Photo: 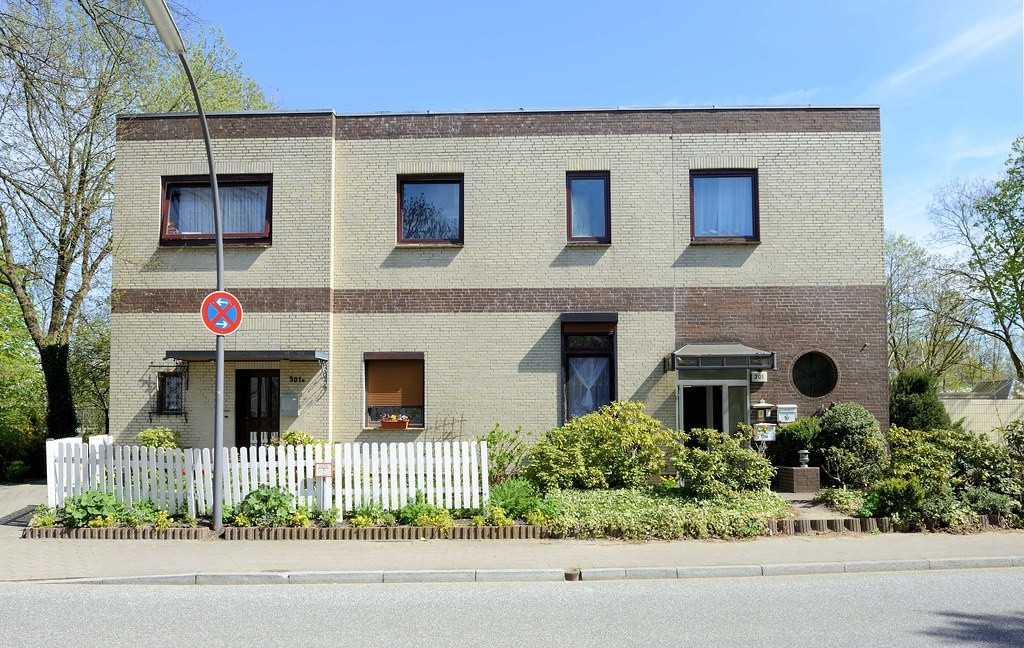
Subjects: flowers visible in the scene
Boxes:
[379,413,409,422]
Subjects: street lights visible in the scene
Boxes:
[142,0,225,534]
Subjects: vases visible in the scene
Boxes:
[381,420,409,430]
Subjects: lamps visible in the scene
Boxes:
[664,357,672,373]
[796,449,811,468]
